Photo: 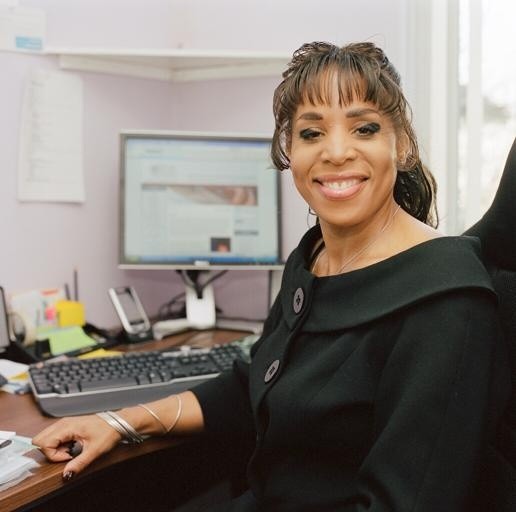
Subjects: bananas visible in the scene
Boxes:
[26,340,250,420]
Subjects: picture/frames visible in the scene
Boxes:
[0,327,252,512]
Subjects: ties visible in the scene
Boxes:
[116,129,287,334]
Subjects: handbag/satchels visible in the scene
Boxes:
[106,411,145,444]
[95,411,142,444]
[137,403,169,435]
[167,394,182,433]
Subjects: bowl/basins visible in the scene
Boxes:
[337,202,403,275]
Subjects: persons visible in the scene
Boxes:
[28,39,516,512]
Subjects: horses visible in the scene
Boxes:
[109,286,151,334]
